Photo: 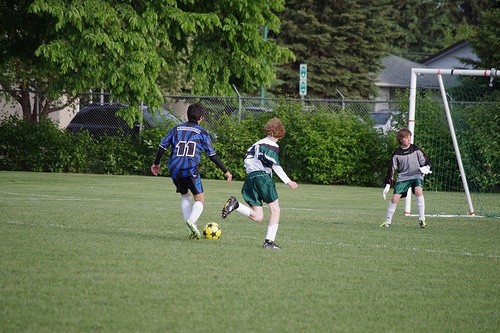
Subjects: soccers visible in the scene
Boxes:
[203,222,221,240]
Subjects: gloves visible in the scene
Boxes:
[418,165,432,175]
[383,184,391,200]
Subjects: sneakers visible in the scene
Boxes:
[221,196,238,220]
[263,239,281,250]
[418,220,426,228]
[379,222,391,227]
[186,219,200,240]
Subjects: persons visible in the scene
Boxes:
[378,128,434,228]
[221,116,298,249]
[150,102,233,240]
